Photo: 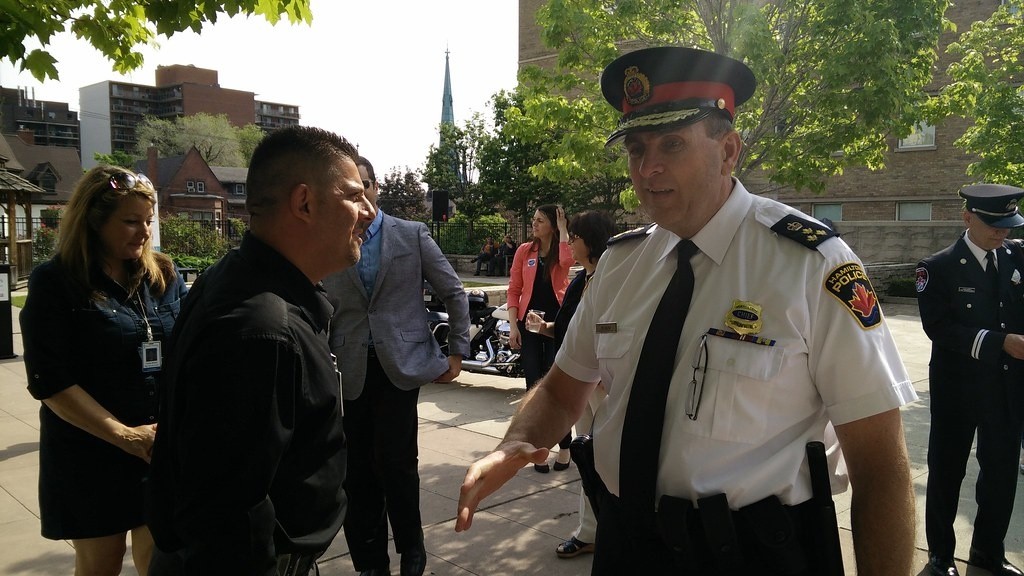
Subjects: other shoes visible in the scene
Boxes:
[473,271,479,275]
[471,258,478,263]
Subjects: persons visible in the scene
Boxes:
[455,46,914,576]
[19,164,190,576]
[322,156,470,576]
[913,184,1024,576]
[554,209,621,559]
[145,128,376,576]
[471,235,516,277]
[506,205,575,473]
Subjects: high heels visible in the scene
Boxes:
[535,462,550,473]
[553,453,571,471]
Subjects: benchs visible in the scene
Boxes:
[477,248,516,276]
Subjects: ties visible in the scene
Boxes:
[985,251,998,289]
[618,240,700,521]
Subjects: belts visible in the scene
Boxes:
[367,345,377,357]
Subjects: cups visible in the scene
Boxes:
[528,310,546,333]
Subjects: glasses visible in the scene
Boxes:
[362,179,374,189]
[685,334,708,420]
[568,233,584,245]
[87,172,154,209]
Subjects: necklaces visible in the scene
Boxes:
[538,249,549,267]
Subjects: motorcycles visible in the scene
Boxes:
[424,287,524,378]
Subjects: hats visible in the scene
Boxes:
[600,47,756,149]
[959,184,1024,228]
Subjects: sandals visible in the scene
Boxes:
[556,536,596,557]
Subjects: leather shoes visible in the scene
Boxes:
[966,556,1024,576]
[358,563,390,576]
[928,546,959,576]
[400,528,426,576]
